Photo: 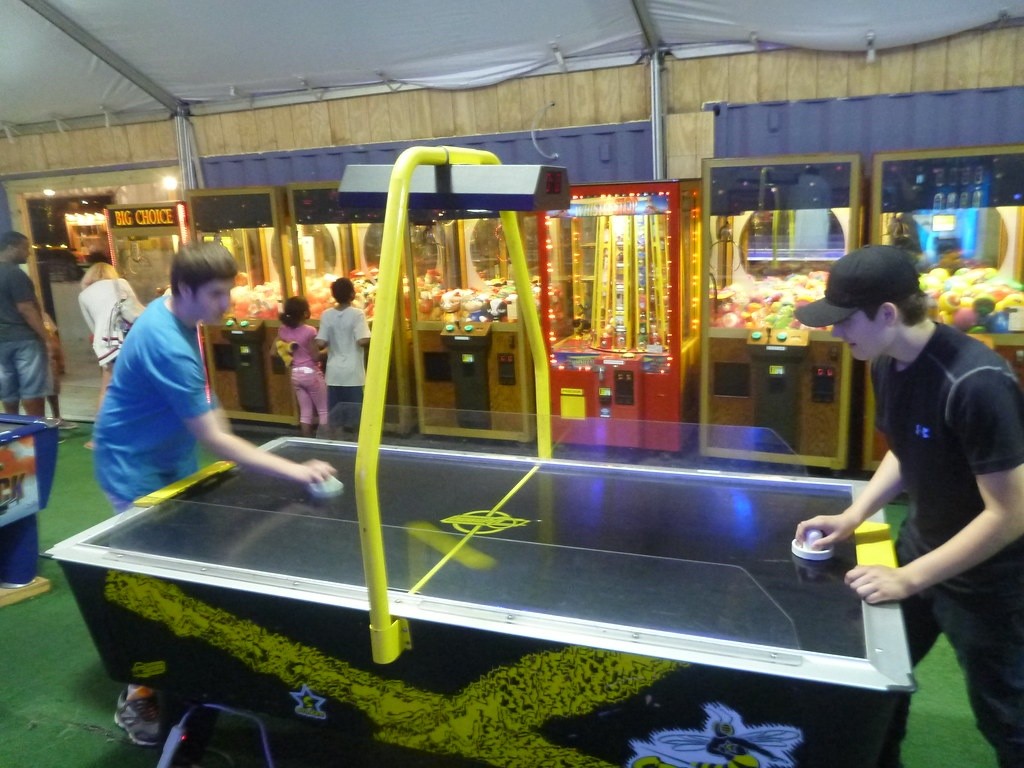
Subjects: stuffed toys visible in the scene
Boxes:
[920,267,1024,332]
[308,267,379,317]
[275,341,299,367]
[715,270,834,332]
[230,281,297,319]
[401,270,541,323]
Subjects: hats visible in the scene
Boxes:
[793,244,921,327]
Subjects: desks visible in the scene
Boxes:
[38,434,918,768]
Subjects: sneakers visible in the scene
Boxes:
[114,688,164,745]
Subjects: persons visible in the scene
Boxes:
[930,237,971,273]
[78,262,136,450]
[93,243,336,746]
[314,277,371,440]
[269,296,329,438]
[794,245,1023,768]
[0,231,61,425]
